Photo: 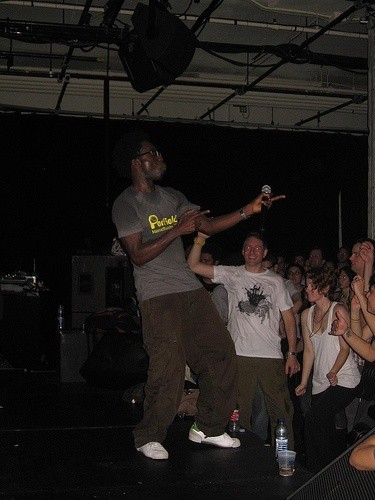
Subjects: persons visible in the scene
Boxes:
[188,232,301,448]
[264,238,375,472]
[96,296,141,334]
[111,138,286,460]
[199,248,245,325]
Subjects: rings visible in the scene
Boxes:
[355,278,357,280]
[269,199,270,201]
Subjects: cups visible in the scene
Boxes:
[277,450,296,477]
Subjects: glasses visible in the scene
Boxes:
[137,148,162,159]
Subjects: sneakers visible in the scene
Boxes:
[137,441,168,460]
[189,421,241,448]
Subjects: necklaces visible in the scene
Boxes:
[313,300,332,324]
[309,300,326,338]
[342,292,347,297]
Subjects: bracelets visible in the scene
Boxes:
[351,315,362,321]
[329,372,336,375]
[240,209,247,219]
[288,352,295,355]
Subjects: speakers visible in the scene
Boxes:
[118,4,198,92]
[70,255,136,332]
[286,426,375,500]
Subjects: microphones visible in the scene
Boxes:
[259,185,271,227]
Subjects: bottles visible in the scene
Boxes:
[275,418,288,462]
[229,406,240,433]
